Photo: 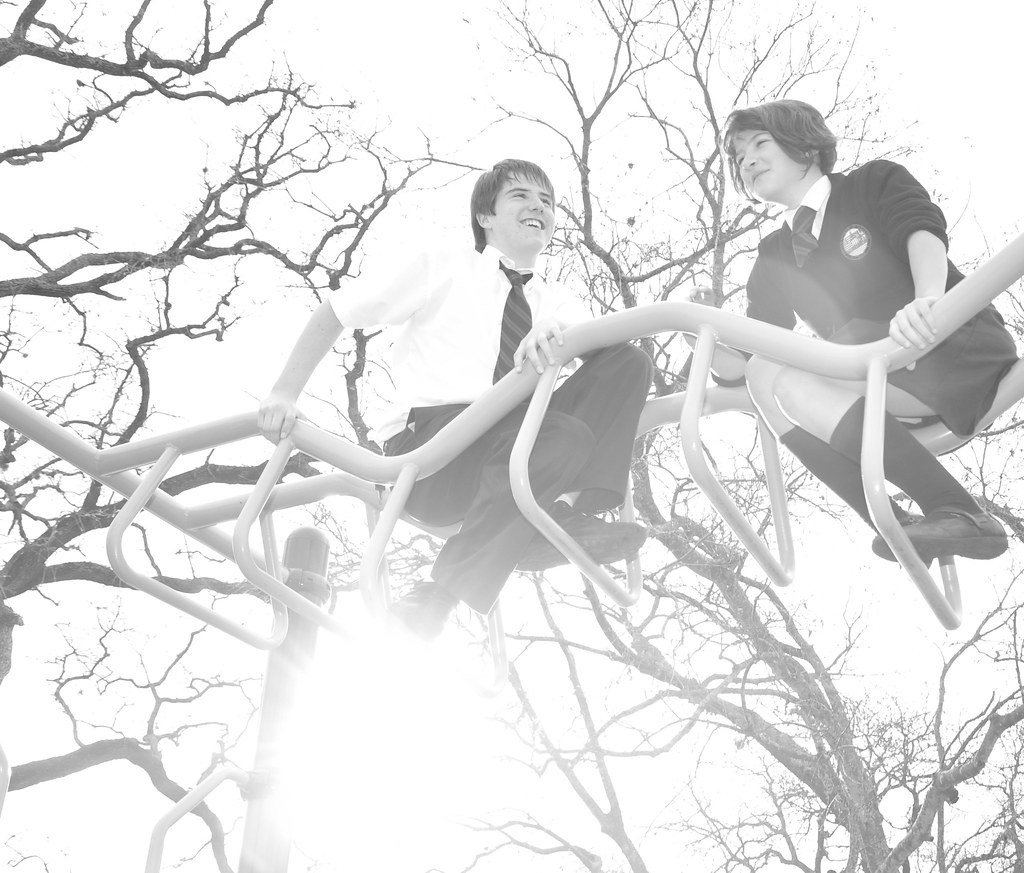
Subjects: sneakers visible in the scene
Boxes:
[516,508,647,571]
[898,511,925,527]
[872,506,1009,564]
[390,581,460,640]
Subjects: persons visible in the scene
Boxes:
[259,159,654,629]
[682,100,1018,566]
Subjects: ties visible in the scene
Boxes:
[790,205,819,268]
[492,260,534,386]
